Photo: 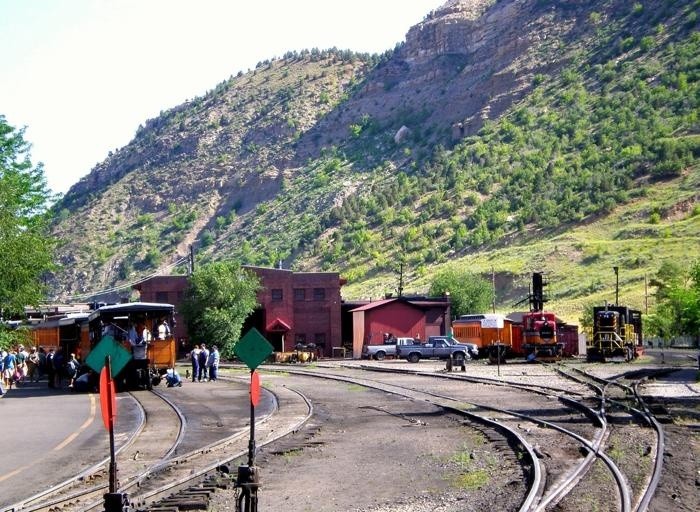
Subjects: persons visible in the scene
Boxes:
[190,345,204,382]
[198,343,209,382]
[208,344,220,382]
[166,368,182,387]
[0,340,94,394]
[100,317,171,347]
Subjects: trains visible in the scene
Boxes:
[451,313,514,356]
[28,299,175,391]
[584,305,643,364]
[521,310,567,363]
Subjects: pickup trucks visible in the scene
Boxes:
[368,334,480,364]
